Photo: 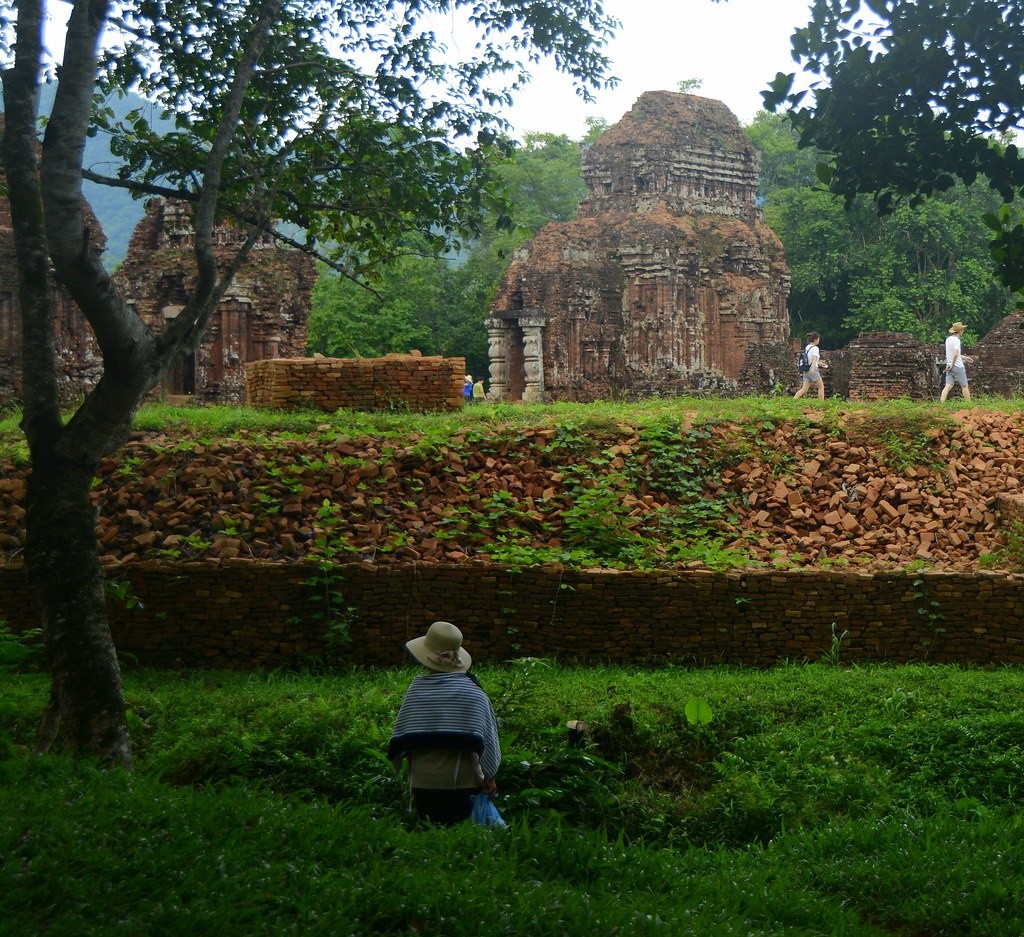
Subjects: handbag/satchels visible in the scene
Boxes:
[472,793,505,829]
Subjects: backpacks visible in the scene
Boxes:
[797,345,814,371]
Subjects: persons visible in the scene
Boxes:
[464,375,474,396]
[472,376,487,400]
[939,322,974,403]
[382,621,502,829]
[793,331,829,400]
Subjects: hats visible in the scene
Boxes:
[464,374,474,385]
[406,621,472,672]
[948,322,969,333]
